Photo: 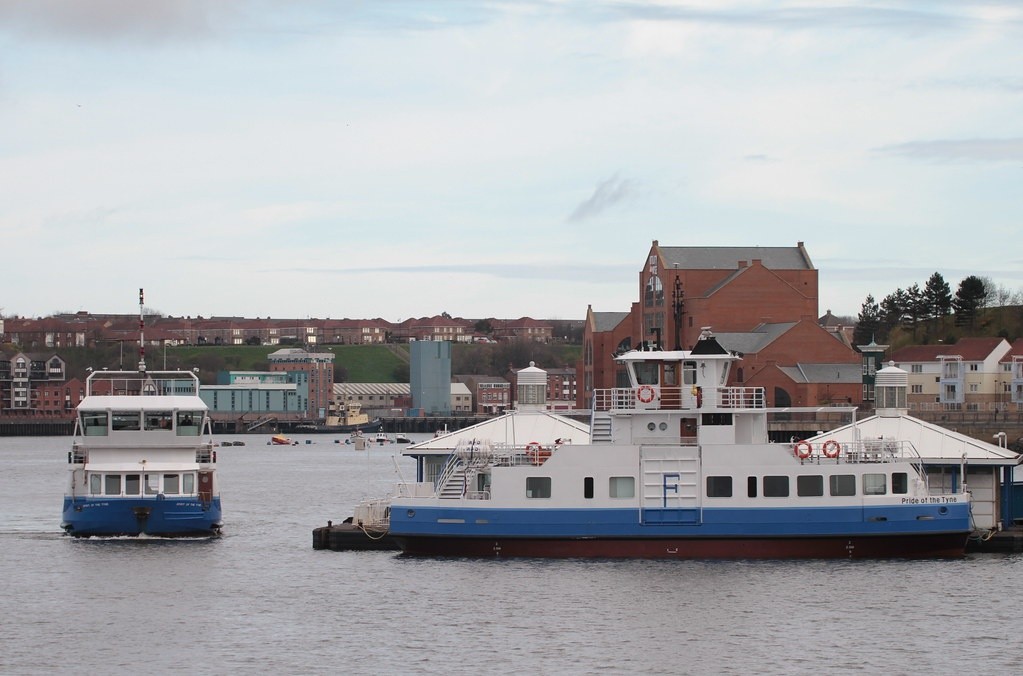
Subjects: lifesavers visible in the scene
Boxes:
[636,385,655,403]
[794,440,813,458]
[822,439,840,457]
[525,441,543,460]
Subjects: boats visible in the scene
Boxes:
[59,288,221,545]
[376,433,387,442]
[313,327,971,560]
[293,401,383,433]
[396,432,411,443]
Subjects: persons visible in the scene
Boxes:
[181,415,192,426]
